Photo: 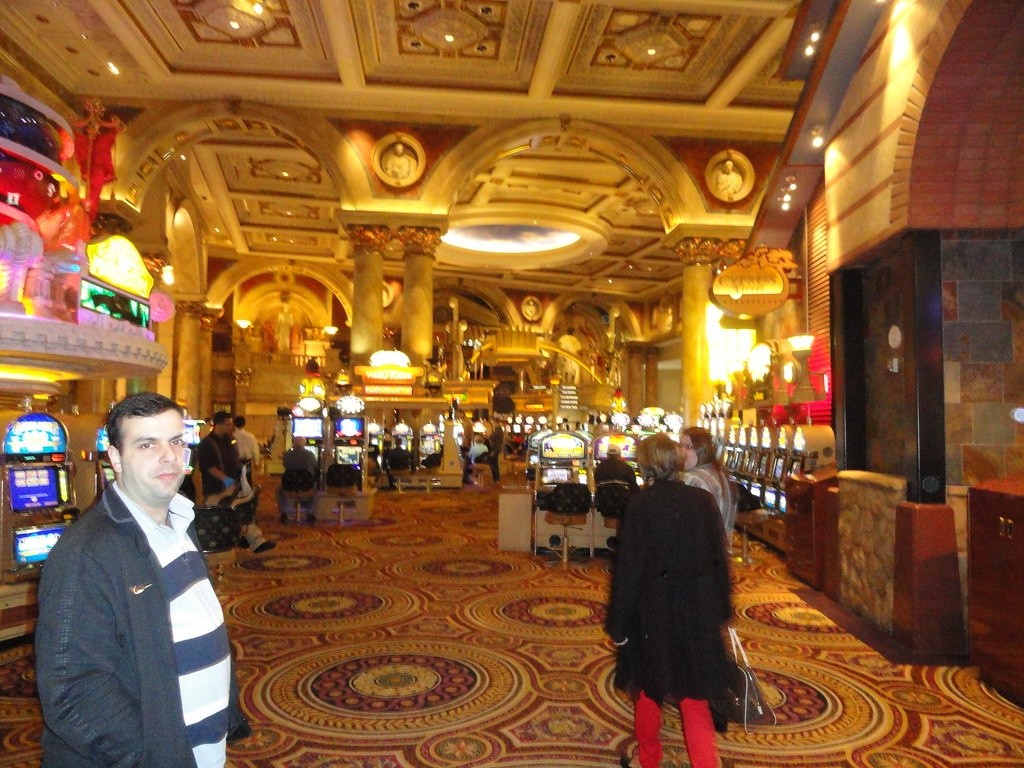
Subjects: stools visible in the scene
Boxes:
[732,482,764,564]
[391,469,407,495]
[470,463,490,488]
[419,453,442,497]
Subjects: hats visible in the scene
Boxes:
[607,445,621,454]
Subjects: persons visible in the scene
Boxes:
[199,411,276,553]
[36,391,252,768]
[606,427,738,768]
[594,445,640,519]
[367,418,523,491]
[280,435,320,523]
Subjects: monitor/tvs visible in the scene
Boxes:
[369,436,442,456]
[8,466,59,511]
[293,417,323,437]
[292,444,320,463]
[540,467,573,484]
[335,418,363,436]
[724,447,802,480]
[335,445,362,464]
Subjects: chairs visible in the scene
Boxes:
[326,464,363,527]
[594,483,630,529]
[536,483,592,571]
[282,467,317,526]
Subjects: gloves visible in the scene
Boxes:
[224,476,237,489]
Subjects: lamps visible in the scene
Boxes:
[142,252,175,285]
[297,375,325,413]
[789,333,815,360]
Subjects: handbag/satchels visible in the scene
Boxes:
[727,627,777,735]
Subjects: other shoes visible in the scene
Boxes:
[281,513,287,522]
[238,536,250,549]
[253,542,276,553]
[620,756,634,768]
[307,514,317,522]
